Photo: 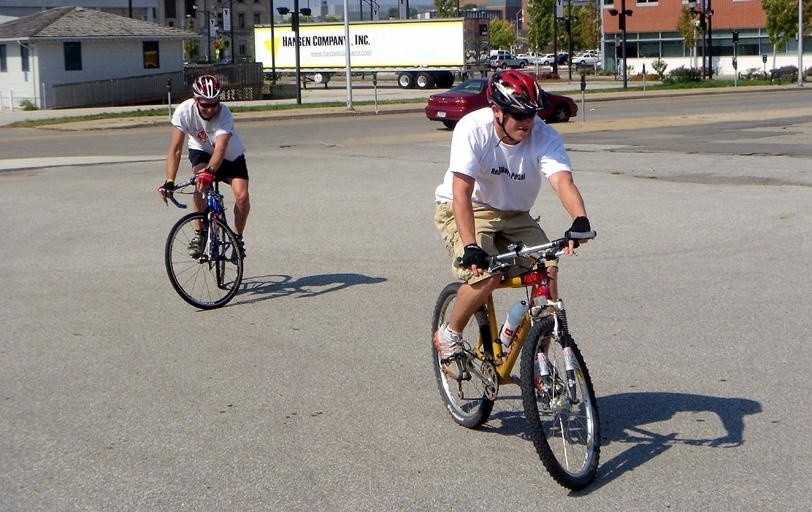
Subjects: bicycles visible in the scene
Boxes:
[158,171,245,309]
[431,232,601,495]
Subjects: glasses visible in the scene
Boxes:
[509,108,540,123]
[198,100,220,109]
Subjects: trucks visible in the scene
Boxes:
[216,17,482,89]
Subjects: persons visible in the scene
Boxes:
[163,74,251,265]
[432,67,592,404]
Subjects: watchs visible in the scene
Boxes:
[206,167,216,173]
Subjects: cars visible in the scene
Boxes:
[489,48,602,75]
[424,76,580,135]
[182,58,212,85]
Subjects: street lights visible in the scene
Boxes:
[688,0,714,82]
[562,0,579,82]
[609,0,634,90]
[277,0,309,104]
[182,1,237,65]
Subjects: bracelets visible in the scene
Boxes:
[167,179,175,184]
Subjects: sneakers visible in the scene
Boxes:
[189,229,209,258]
[433,322,473,381]
[535,355,563,396]
[230,234,246,263]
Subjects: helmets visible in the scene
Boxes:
[486,70,544,114]
[192,74,223,102]
